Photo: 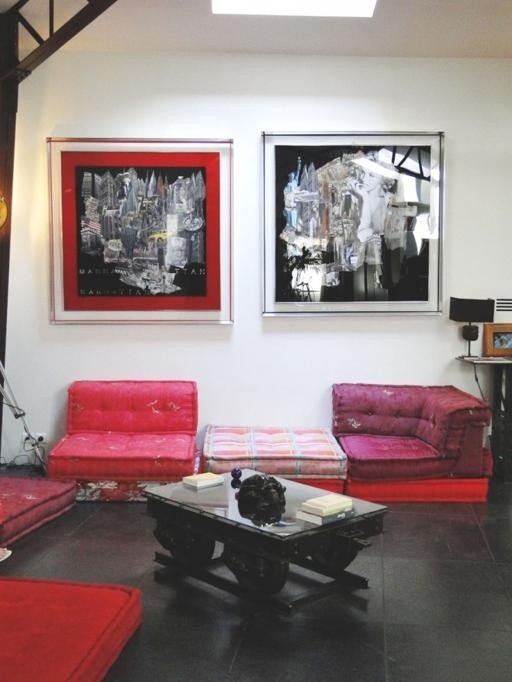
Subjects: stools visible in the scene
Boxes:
[202,427,348,495]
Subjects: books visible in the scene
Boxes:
[463,356,512,364]
[182,472,224,488]
[292,494,355,524]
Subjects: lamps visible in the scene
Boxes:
[449,296,494,358]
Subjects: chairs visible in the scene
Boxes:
[334,382,492,503]
[46,380,199,503]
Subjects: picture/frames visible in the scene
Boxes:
[48,138,235,324]
[482,323,511,358]
[262,132,444,314]
